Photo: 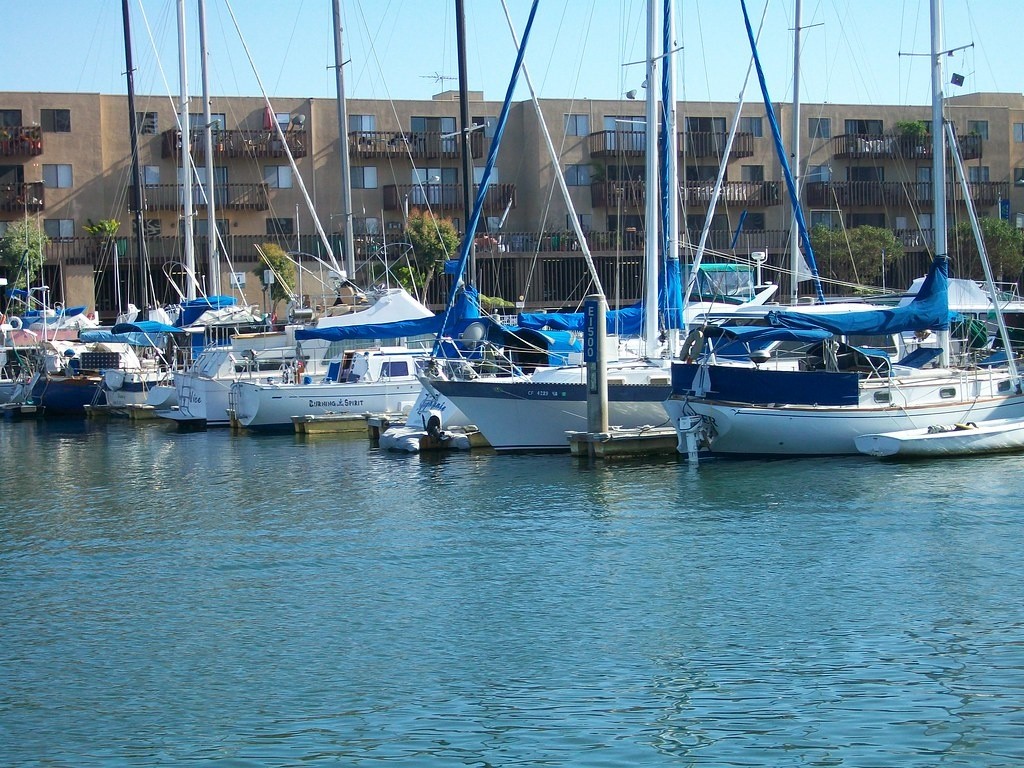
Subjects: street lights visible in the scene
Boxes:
[402,175,438,252]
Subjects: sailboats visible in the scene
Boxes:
[0,0,1024,459]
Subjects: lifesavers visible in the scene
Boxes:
[5,315,23,331]
[678,330,704,363]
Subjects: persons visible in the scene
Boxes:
[421,414,452,442]
